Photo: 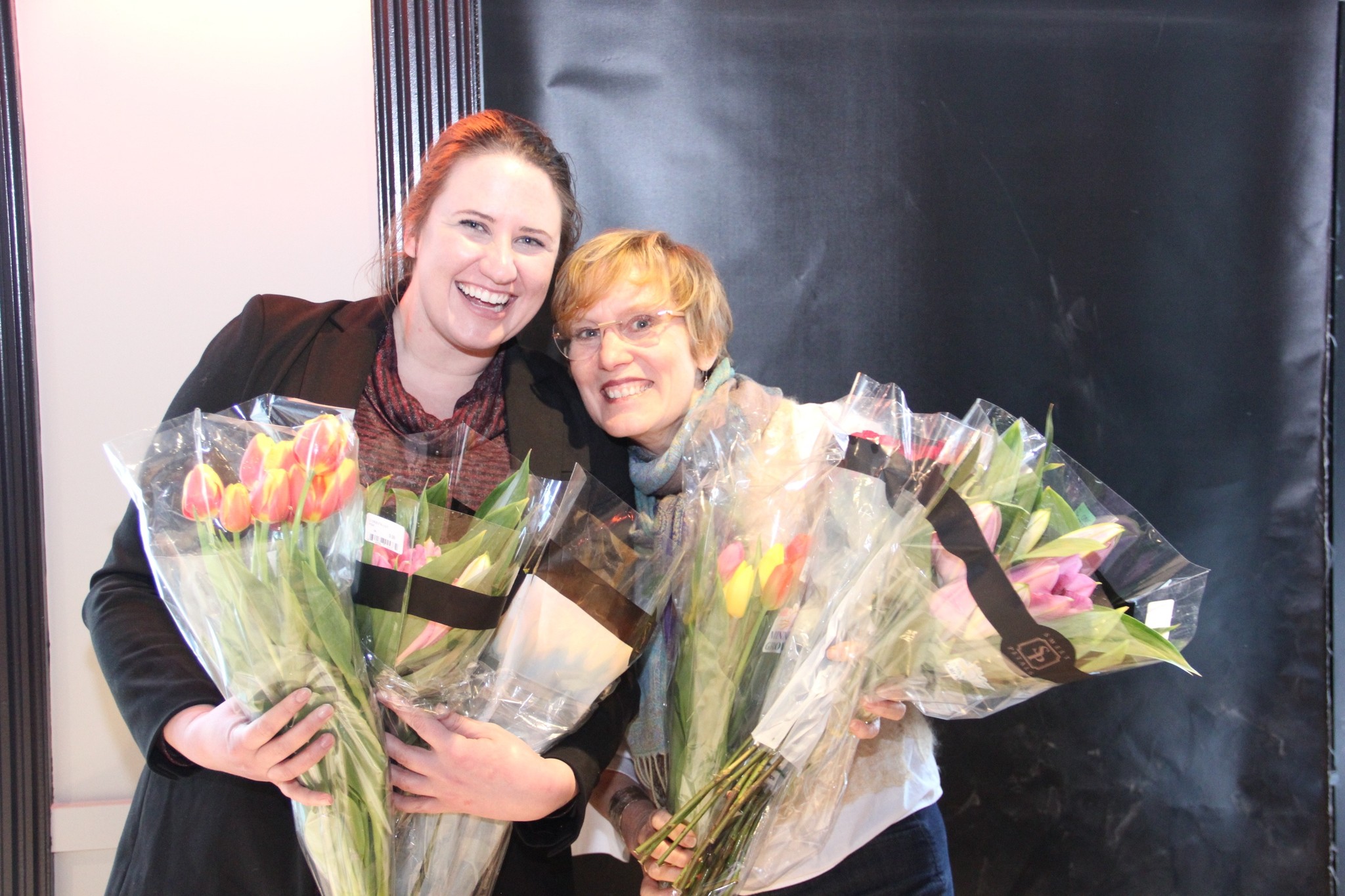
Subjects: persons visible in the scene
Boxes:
[81,111,643,896]
[551,230,955,896]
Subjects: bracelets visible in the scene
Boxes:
[606,785,651,840]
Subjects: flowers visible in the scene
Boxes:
[99,368,1210,896]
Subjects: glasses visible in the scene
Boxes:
[552,305,688,362]
